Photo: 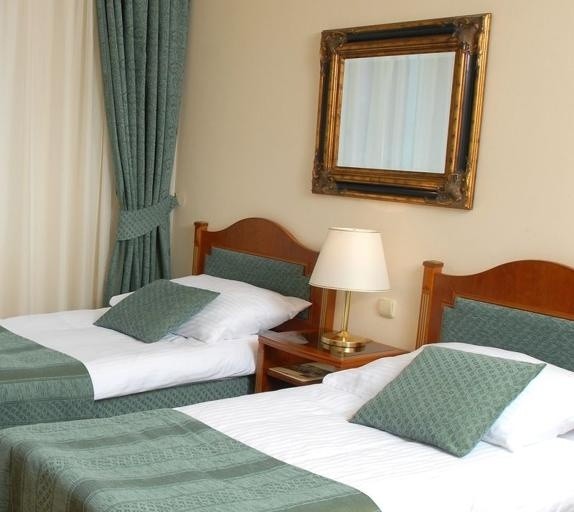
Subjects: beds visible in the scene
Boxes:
[0,215,337,431]
[0,254,574,512]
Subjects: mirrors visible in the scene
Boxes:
[310,11,494,212]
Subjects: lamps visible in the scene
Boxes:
[307,225,393,350]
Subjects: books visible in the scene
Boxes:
[268,362,336,381]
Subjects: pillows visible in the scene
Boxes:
[346,346,549,460]
[91,277,222,345]
[108,272,314,345]
[320,339,574,455]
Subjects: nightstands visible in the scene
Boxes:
[252,328,412,395]
